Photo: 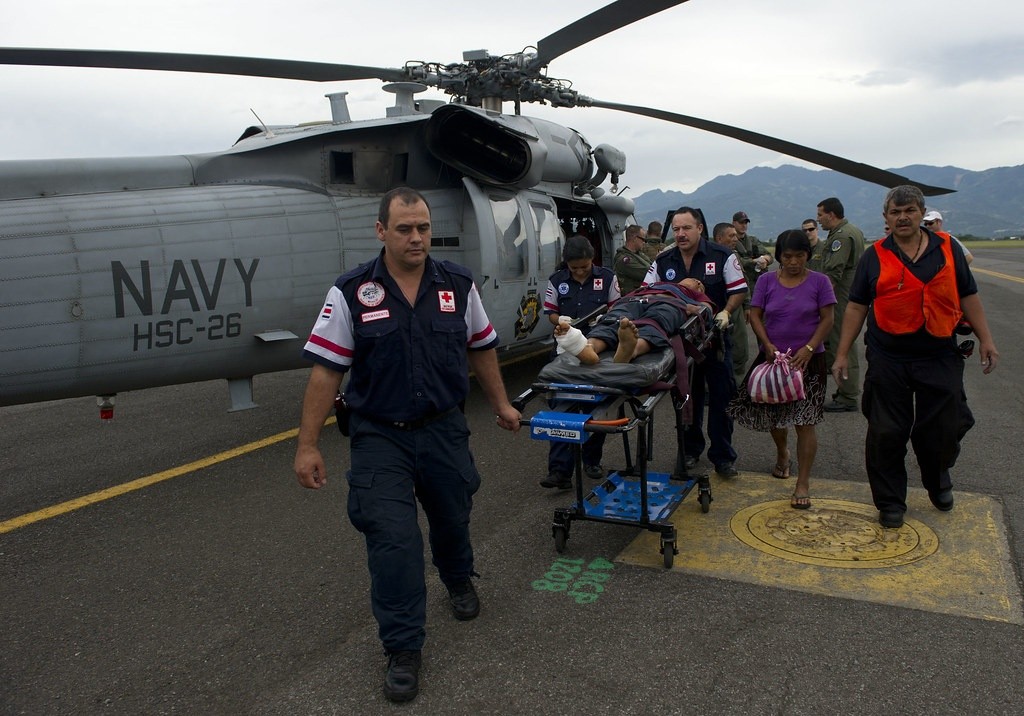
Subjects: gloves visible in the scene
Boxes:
[558,315,573,325]
[715,310,730,330]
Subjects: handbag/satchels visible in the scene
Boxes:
[748,347,805,403]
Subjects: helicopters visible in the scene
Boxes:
[0,0,961,422]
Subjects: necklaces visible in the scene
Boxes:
[778,269,806,286]
[893,230,923,289]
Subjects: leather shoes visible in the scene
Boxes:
[879,510,904,528]
[540,473,572,490]
[714,460,738,476]
[449,575,480,621]
[583,463,602,479]
[383,648,421,703]
[928,485,953,511]
[684,454,699,468]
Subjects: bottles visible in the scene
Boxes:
[755,256,765,272]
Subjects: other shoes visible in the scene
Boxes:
[822,400,858,412]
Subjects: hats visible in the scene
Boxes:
[922,210,943,221]
[733,211,750,223]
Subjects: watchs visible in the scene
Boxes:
[807,345,815,354]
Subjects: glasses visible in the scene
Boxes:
[635,235,645,242]
[885,227,890,230]
[924,220,938,226]
[802,226,816,233]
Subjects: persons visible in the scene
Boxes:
[725,230,837,509]
[802,219,825,272]
[293,187,523,701]
[613,222,667,300]
[553,277,717,366]
[538,236,621,491]
[883,210,973,267]
[713,222,751,385]
[817,198,865,414]
[731,211,775,351]
[830,184,998,528]
[640,205,749,478]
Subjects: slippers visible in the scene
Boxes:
[791,494,811,509]
[772,458,792,478]
